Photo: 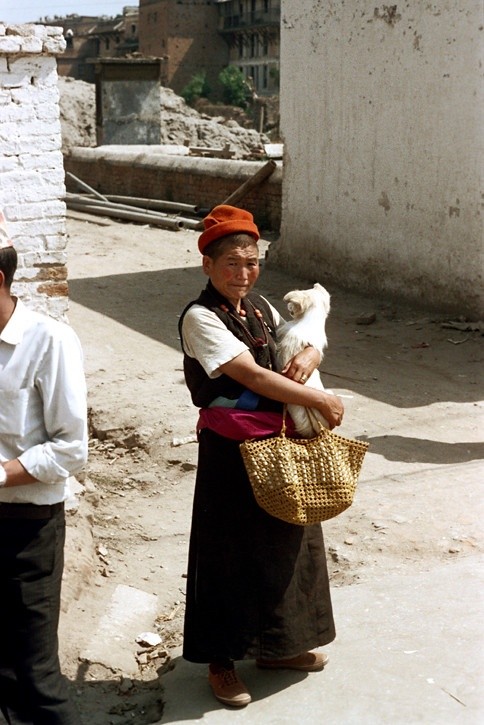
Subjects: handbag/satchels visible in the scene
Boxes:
[239,397,369,526]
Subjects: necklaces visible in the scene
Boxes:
[220,297,268,348]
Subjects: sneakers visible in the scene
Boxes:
[207,666,250,707]
[255,651,328,672]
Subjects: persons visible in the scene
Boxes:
[177,205,344,706]
[0,212,89,725]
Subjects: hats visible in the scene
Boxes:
[197,203,261,254]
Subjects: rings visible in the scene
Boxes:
[301,374,307,381]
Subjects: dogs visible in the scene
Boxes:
[274,283,330,438]
